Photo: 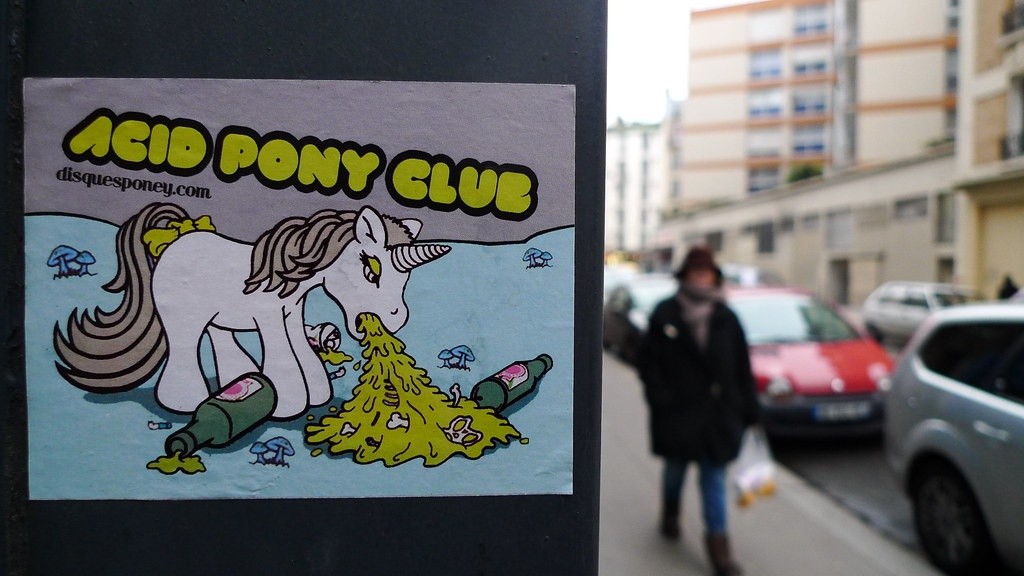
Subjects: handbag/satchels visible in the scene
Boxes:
[732,427,782,510]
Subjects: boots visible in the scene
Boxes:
[704,533,744,576]
[657,499,682,542]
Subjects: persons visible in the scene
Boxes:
[638,247,766,576]
[998,276,1018,299]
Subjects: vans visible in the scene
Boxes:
[719,285,896,461]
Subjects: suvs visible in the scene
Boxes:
[863,283,971,348]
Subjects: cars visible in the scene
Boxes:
[886,303,1023,576]
[604,271,679,366]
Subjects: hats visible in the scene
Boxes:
[673,246,725,290]
